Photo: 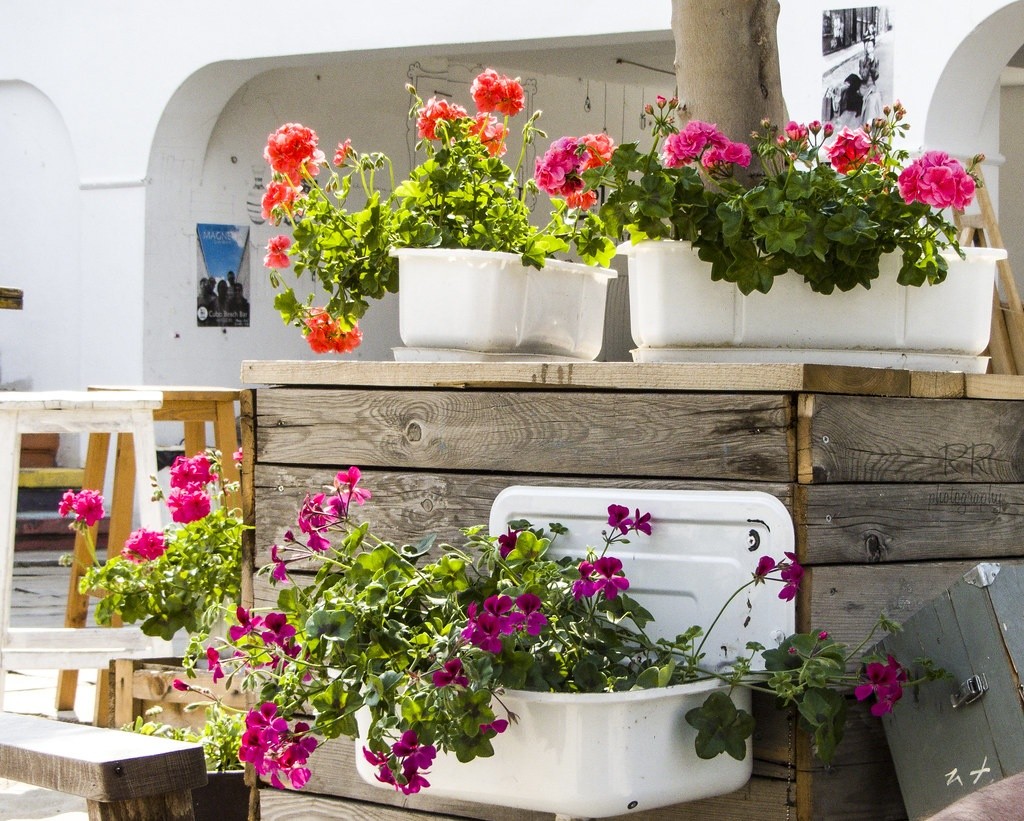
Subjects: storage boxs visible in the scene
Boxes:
[239,355,1024,820]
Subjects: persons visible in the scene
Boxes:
[829,12,888,128]
[198,270,250,324]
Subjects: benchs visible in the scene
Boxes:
[0,717,208,821]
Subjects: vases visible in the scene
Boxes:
[354,483,796,819]
[387,248,620,364]
[613,238,1007,373]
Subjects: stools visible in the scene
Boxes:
[0,385,242,732]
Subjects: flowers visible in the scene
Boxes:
[170,461,957,798]
[58,445,243,639]
[260,66,619,353]
[535,94,986,295]
